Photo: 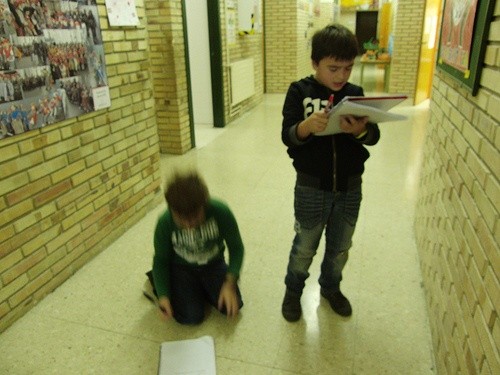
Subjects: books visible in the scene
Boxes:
[157,334,217,375]
[315,94,408,136]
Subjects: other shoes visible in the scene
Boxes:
[281,288,303,322]
[145,269,162,296]
[320,285,352,317]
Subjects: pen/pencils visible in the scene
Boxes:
[325,94,334,113]
[142,292,167,316]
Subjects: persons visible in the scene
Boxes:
[141,167,245,326]
[0,1,106,137]
[281,23,380,323]
